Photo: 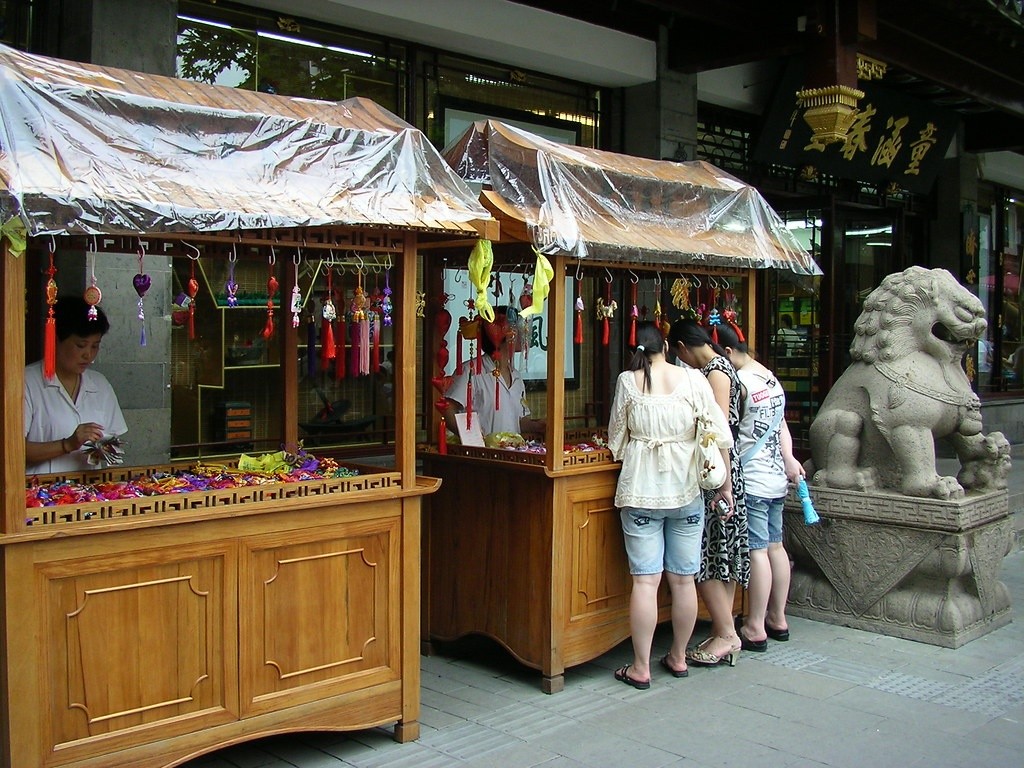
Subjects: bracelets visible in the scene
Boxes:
[62,438,70,454]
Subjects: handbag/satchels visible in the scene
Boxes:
[686,368,727,490]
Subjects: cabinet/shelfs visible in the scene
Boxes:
[417,442,750,694]
[0,473,441,768]
[775,356,820,425]
[172,17,394,461]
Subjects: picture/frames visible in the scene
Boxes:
[434,93,583,393]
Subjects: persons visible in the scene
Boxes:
[609,321,733,690]
[445,306,547,438]
[978,341,1017,382]
[380,351,394,375]
[667,320,750,667]
[771,315,802,355]
[710,324,805,651]
[25,295,128,473]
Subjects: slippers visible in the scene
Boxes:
[736,626,767,652]
[660,651,688,678]
[614,665,650,689]
[764,618,790,641]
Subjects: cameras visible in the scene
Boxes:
[715,500,729,516]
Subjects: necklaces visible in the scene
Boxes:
[56,373,78,399]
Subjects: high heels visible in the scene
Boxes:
[685,631,741,667]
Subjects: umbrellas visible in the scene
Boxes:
[796,475,819,525]
